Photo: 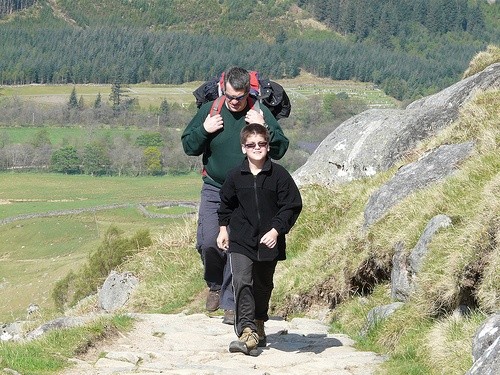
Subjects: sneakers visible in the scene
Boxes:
[223,309,236,324]
[228,318,268,356]
[206,289,222,311]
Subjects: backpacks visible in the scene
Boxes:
[206,67,262,134]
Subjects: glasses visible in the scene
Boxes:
[224,92,248,100]
[244,141,268,148]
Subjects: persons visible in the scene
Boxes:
[216,123,304,356]
[180,66,290,325]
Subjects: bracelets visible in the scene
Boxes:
[262,122,269,129]
